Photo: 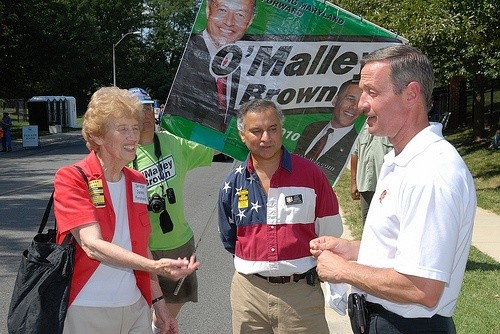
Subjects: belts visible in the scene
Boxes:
[253,266,317,283]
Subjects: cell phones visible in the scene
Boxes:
[353,295,365,333]
[166,187,176,204]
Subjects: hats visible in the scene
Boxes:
[127,88,154,104]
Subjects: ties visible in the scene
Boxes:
[305,128,335,161]
[216,58,227,115]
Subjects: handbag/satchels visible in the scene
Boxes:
[7,165,88,334]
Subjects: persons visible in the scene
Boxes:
[217,99,344,334]
[0,111,12,152]
[164,1,256,132]
[54,86,201,334]
[309,46,477,334]
[469,92,500,155]
[123,88,223,334]
[350,113,395,231]
[293,79,367,181]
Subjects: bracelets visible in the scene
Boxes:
[152,295,164,304]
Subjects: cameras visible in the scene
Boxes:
[147,193,166,213]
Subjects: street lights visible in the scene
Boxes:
[113,32,141,87]
